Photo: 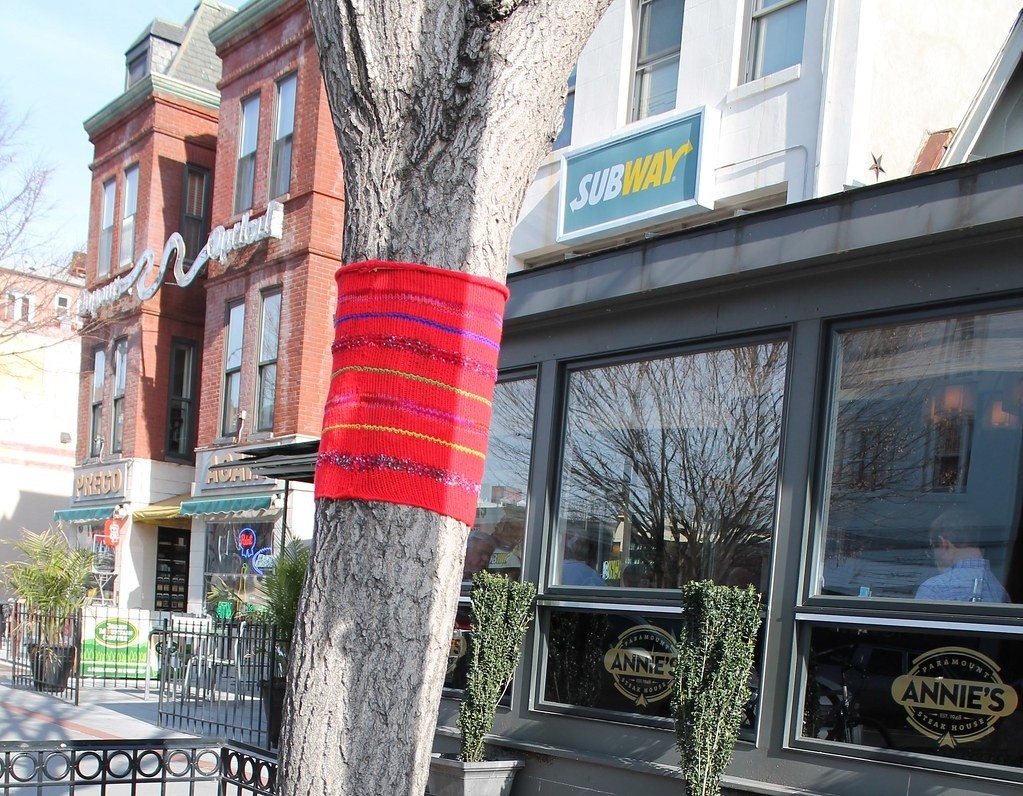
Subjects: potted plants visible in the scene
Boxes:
[1,515,114,693]
[206,518,314,749]
[425,569,539,796]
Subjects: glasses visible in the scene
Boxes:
[924,541,935,559]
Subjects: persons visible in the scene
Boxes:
[463,531,498,575]
[559,525,607,586]
[914,508,1012,604]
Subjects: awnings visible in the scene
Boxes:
[178,494,281,514]
[53,506,119,523]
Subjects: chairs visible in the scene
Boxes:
[183,620,247,708]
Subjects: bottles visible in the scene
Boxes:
[969,577,986,603]
